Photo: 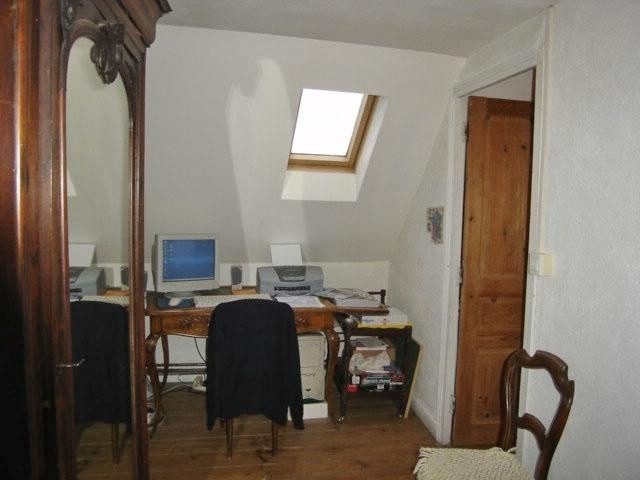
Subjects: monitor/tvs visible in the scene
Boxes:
[151,233,221,299]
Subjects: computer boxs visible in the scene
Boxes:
[297,333,325,402]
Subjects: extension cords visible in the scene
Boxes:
[192,382,206,392]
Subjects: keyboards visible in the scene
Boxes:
[193,294,273,309]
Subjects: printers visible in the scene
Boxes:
[69,266,106,299]
[257,265,324,299]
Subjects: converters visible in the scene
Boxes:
[192,377,202,386]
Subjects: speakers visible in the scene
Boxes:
[231,265,243,290]
[120,264,130,291]
[144,271,148,298]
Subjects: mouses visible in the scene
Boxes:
[168,298,183,307]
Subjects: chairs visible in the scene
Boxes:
[412,348,574,480]
[209,297,291,459]
[71,300,129,467]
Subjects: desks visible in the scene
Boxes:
[68,288,130,308]
[144,291,344,437]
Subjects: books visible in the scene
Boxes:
[354,335,388,352]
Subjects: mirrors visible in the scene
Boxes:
[58,19,136,480]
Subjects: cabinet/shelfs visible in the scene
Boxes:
[335,288,412,420]
